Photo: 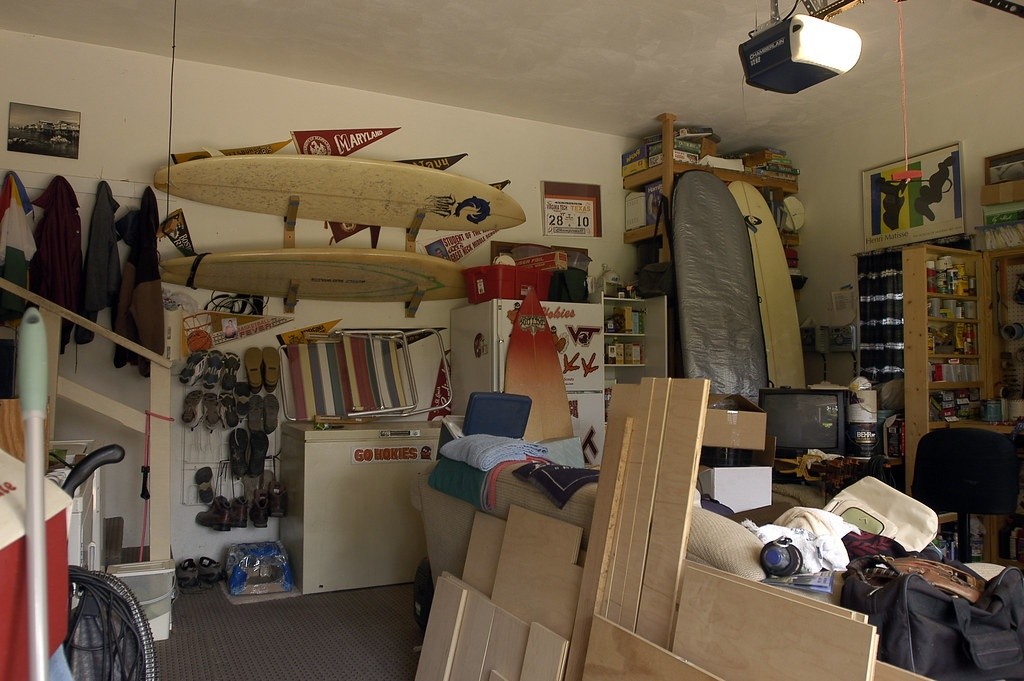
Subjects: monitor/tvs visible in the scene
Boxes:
[758,387,848,457]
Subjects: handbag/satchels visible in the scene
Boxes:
[638,261,675,298]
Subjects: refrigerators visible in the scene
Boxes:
[279,421,443,595]
[450,299,605,469]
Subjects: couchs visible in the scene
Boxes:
[409,463,844,668]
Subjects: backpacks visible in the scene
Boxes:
[842,552,1023,681]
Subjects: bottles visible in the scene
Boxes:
[930,518,1024,564]
[612,336,618,346]
[761,536,833,578]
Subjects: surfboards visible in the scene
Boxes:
[158,247,468,303]
[504,286,574,443]
[154,154,527,232]
[670,170,769,406]
[727,180,806,389]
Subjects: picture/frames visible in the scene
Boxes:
[985,148,1024,185]
[8,102,81,159]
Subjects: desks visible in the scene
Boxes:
[775,458,902,480]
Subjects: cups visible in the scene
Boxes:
[943,300,957,318]
[929,297,940,317]
[928,362,979,381]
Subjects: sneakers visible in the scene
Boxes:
[176,556,224,586]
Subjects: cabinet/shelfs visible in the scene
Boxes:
[591,290,668,426]
[902,243,1024,571]
[623,112,801,301]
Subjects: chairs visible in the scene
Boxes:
[278,328,452,422]
[911,428,1020,563]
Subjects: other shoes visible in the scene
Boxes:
[178,347,287,531]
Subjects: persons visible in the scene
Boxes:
[226,320,235,337]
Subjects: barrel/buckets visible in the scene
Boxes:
[846,422,881,457]
[877,410,904,459]
[847,390,877,422]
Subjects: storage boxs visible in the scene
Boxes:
[0,448,74,681]
[105,559,175,642]
[622,127,799,178]
[975,220,1024,250]
[702,393,776,513]
[462,244,593,306]
[626,181,664,231]
[536,437,585,468]
[980,179,1024,206]
[437,392,532,462]
